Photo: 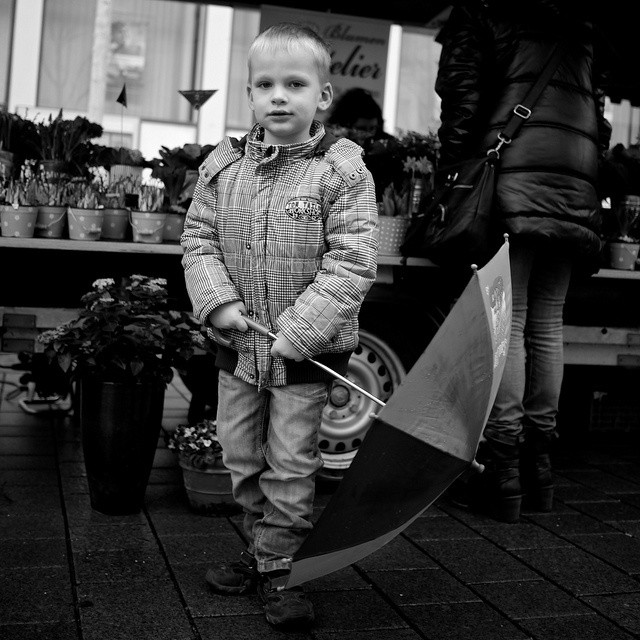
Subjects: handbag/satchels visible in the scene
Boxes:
[427,148,496,267]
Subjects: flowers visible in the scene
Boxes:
[128,176,166,211]
[168,418,224,469]
[401,156,434,179]
[34,273,209,380]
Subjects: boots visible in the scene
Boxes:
[465,447,522,524]
[524,452,555,513]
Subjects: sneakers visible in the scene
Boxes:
[205,551,257,593]
[258,570,314,629]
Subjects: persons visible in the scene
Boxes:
[428,0,607,461]
[178,18,380,631]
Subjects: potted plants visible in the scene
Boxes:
[0,177,38,238]
[606,195,640,270]
[104,145,147,182]
[36,170,66,238]
[27,106,102,172]
[151,144,196,242]
[101,172,132,240]
[0,108,25,177]
[376,181,413,256]
[66,177,106,241]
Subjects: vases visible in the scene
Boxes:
[32,380,207,515]
[408,178,428,213]
[179,461,241,506]
[132,211,167,244]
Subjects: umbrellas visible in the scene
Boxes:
[195,231,516,593]
